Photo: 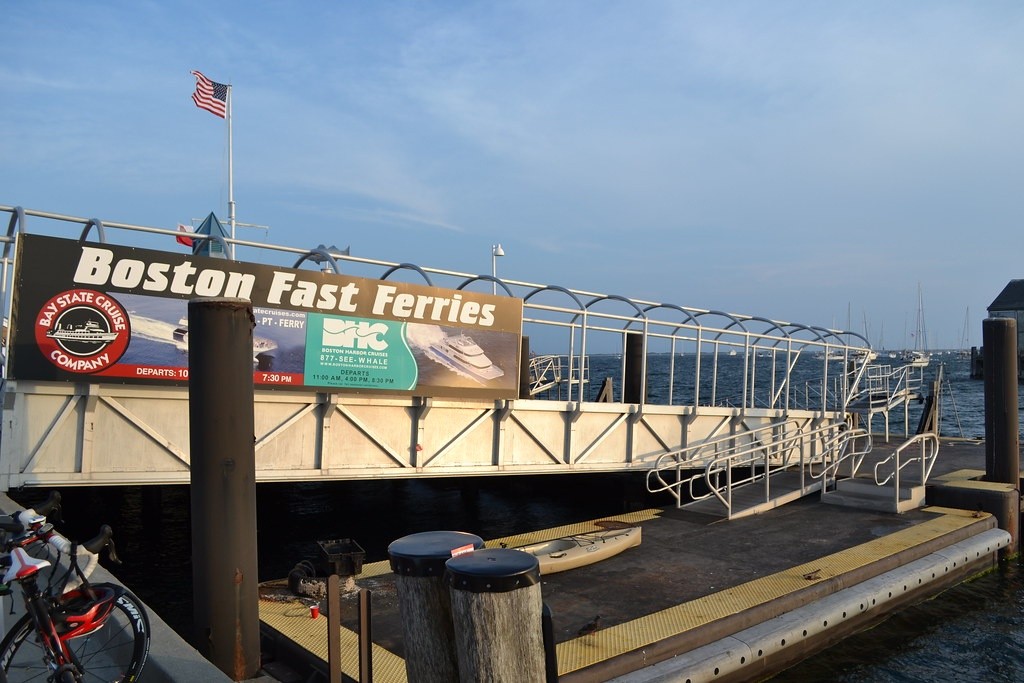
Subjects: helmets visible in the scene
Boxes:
[35,582,127,644]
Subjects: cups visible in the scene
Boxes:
[310,605,319,618]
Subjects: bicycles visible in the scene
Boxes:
[1,491,151,683]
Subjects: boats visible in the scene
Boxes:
[508,526,641,574]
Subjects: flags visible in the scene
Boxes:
[190,70,230,120]
